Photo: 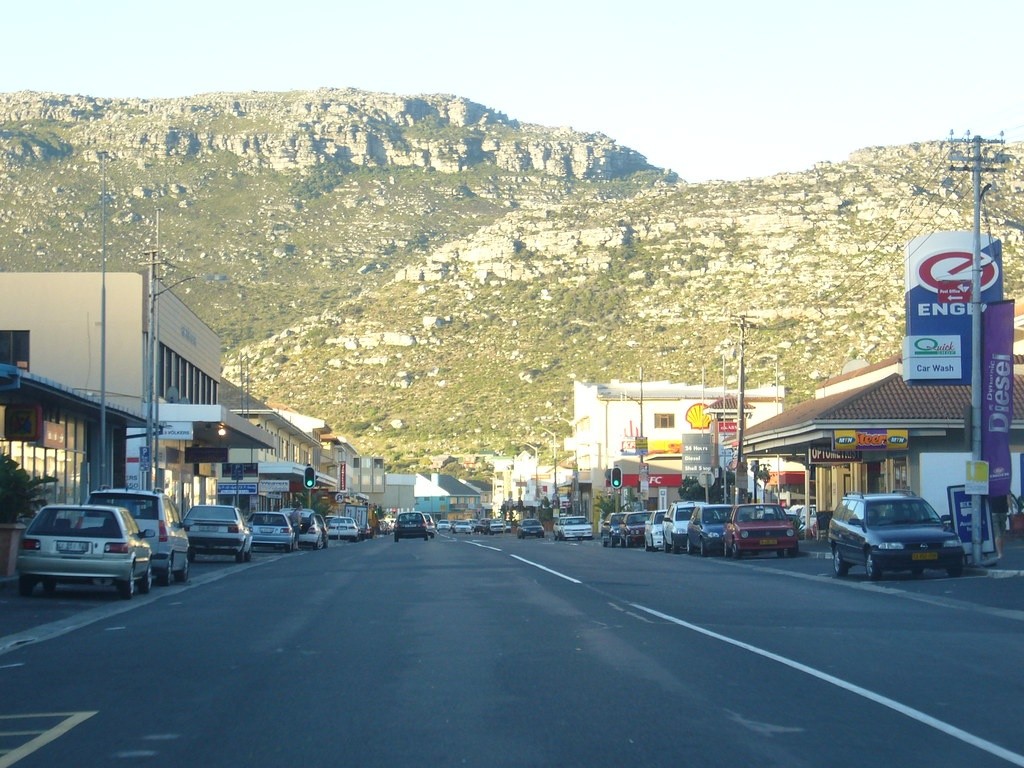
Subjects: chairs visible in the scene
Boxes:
[102,517,119,535]
[54,518,72,533]
[763,513,775,519]
[401,516,406,521]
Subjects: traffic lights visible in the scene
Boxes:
[303,466,315,490]
[610,467,622,489]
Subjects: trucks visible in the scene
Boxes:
[345,504,368,541]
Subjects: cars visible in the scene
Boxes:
[316,513,329,549]
[437,499,800,560]
[827,488,966,581]
[85,484,192,586]
[16,503,153,600]
[393,511,429,542]
[327,516,359,543]
[184,505,253,564]
[246,511,295,553]
[278,507,325,550]
[422,513,435,538]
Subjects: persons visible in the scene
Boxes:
[292,504,303,551]
[989,494,1011,559]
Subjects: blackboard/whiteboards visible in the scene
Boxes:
[816,511,833,531]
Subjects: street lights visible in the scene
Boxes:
[144,274,228,489]
[511,440,539,496]
[528,424,558,493]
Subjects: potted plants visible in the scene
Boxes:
[0,454,60,577]
